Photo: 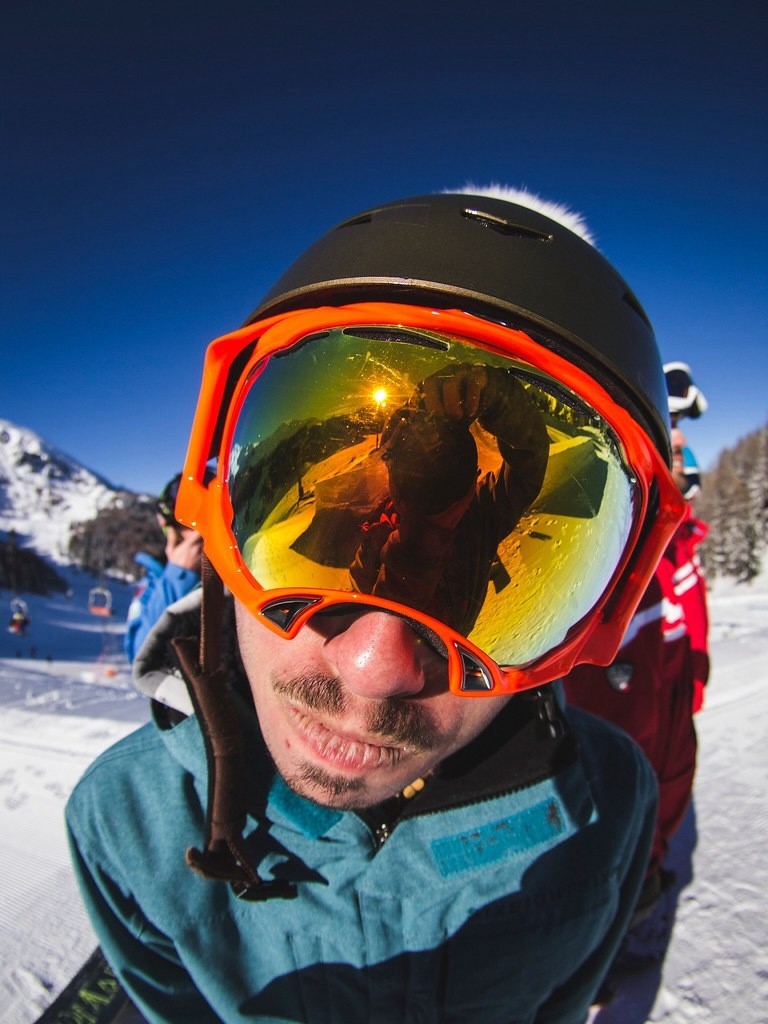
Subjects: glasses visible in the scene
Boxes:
[662,362,708,420]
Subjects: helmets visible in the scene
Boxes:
[156,467,218,527]
[169,191,692,698]
[379,400,482,517]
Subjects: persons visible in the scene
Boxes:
[63,189,696,1024]
[121,361,711,976]
